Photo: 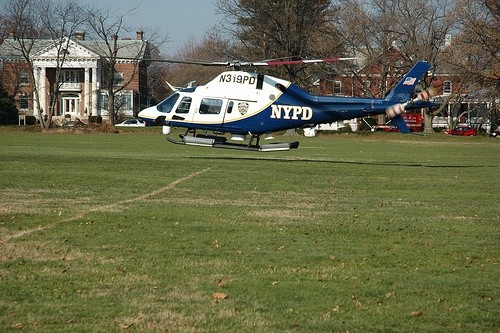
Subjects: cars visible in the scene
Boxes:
[444,126,477,138]
[113,118,147,128]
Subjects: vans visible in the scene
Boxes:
[374,113,427,133]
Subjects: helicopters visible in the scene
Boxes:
[95,51,442,152]
